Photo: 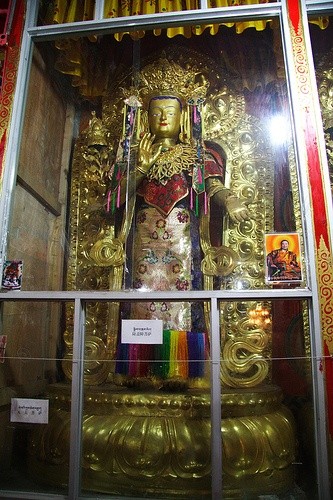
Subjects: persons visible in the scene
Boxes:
[94,58,252,392]
